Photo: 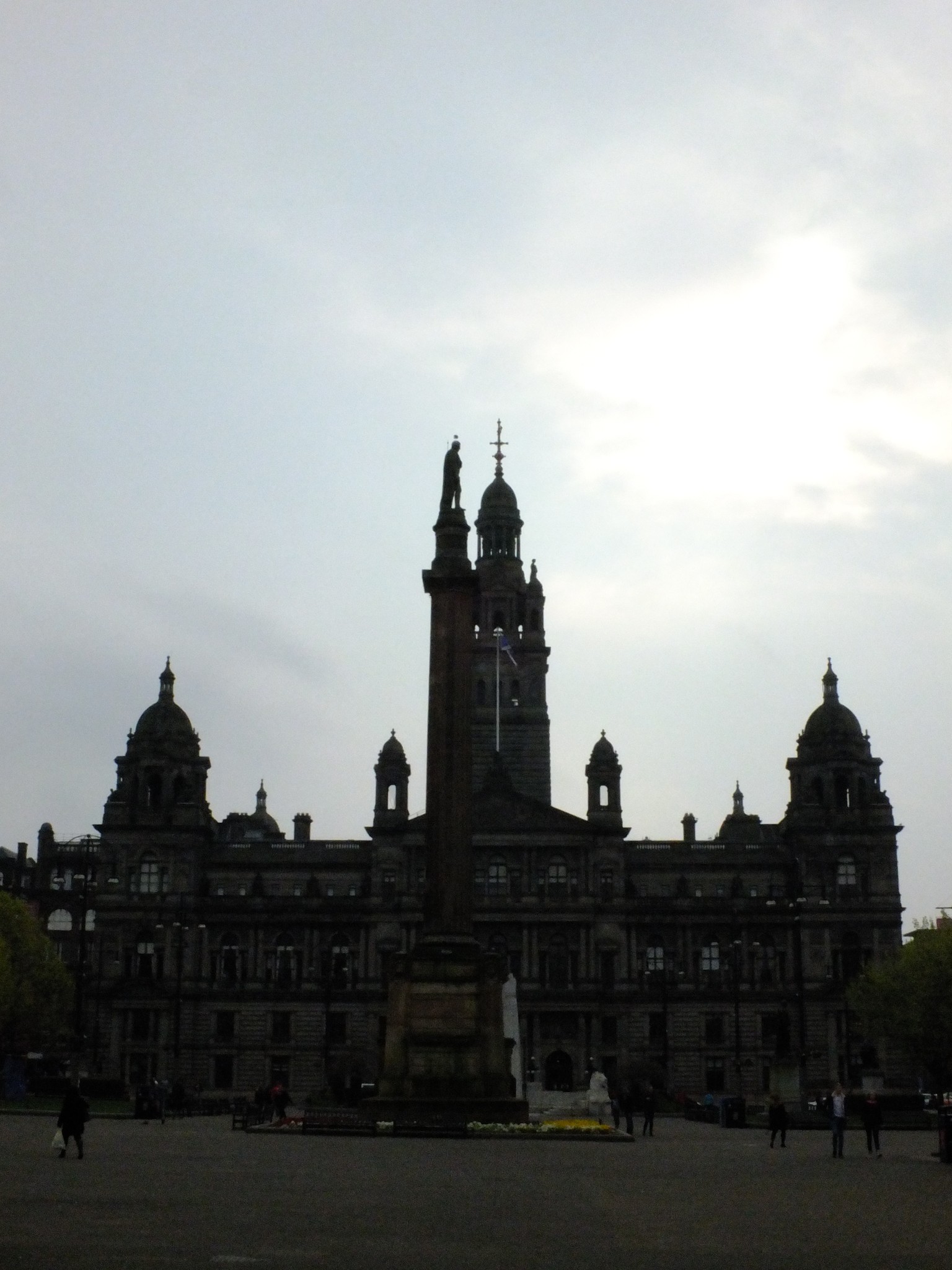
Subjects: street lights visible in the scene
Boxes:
[646,930,668,1104]
[137,924,156,1085]
[72,902,96,1088]
[701,935,721,1099]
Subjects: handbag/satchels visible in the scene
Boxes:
[51,1128,66,1150]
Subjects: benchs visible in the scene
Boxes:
[179,1097,251,1117]
[231,1105,273,1131]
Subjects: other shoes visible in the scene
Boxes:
[781,1144,787,1148]
[932,1149,940,1156]
[875,1149,881,1155]
[144,1120,149,1124]
[643,1130,646,1135]
[649,1131,653,1137]
[78,1152,84,1159]
[58,1151,66,1158]
[162,1120,167,1126]
[770,1142,773,1148]
[939,1157,952,1163]
[867,1146,873,1154]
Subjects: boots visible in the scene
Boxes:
[832,1150,843,1158]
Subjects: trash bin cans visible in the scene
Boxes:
[936,1106,952,1164]
[134,1087,161,1118]
[719,1097,746,1128]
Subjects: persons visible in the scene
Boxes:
[825,1082,853,1160]
[255,1082,286,1123]
[610,1079,635,1136]
[641,1085,656,1137]
[57,1087,89,1160]
[767,1092,789,1149]
[861,1088,883,1157]
